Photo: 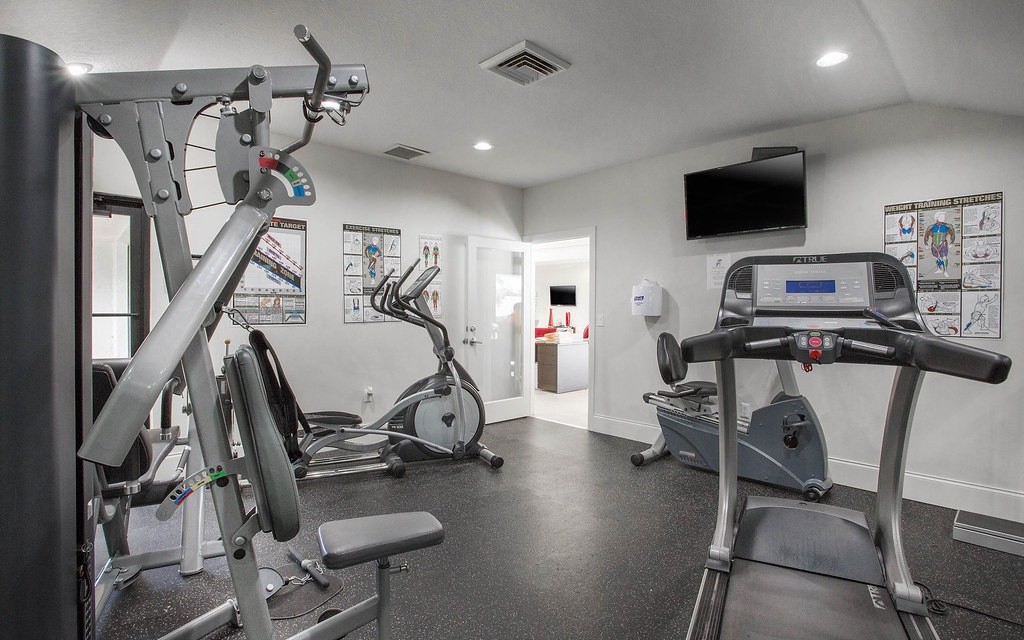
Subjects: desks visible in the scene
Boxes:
[536,342,589,394]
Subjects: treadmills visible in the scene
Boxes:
[679,249,1016,639]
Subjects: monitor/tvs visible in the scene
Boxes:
[550,286,576,306]
[684,149,807,240]
